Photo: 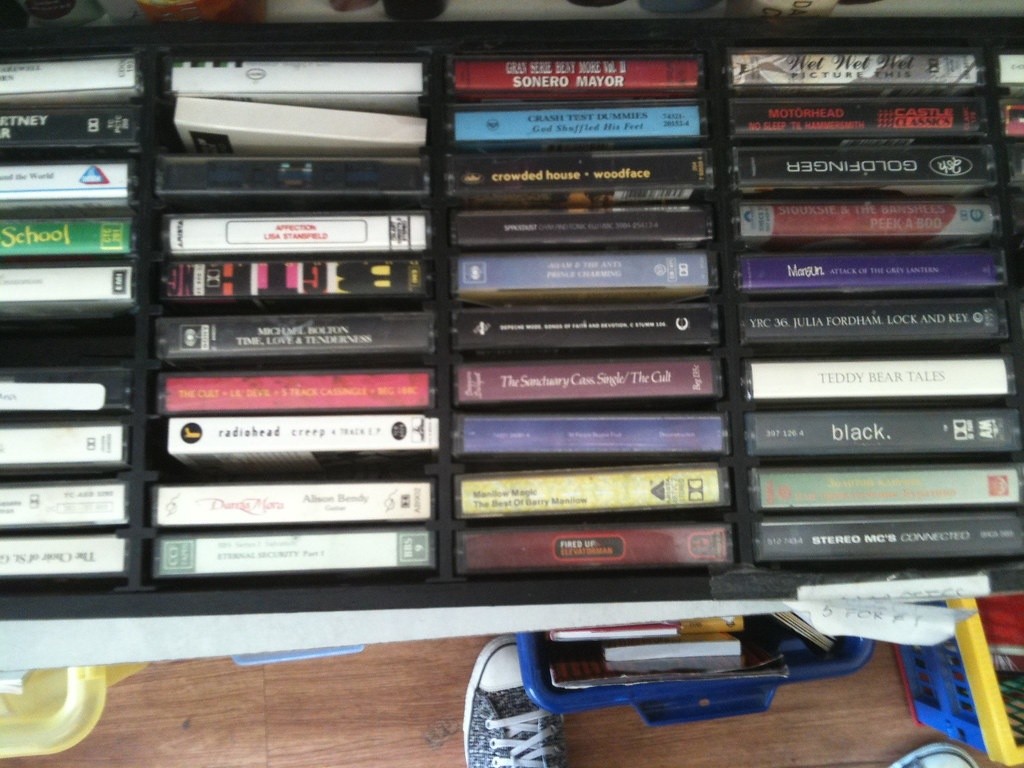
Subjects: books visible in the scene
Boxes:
[0,53,1024,578]
[543,611,834,689]
[1,669,33,694]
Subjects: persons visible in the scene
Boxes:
[463,635,980,768]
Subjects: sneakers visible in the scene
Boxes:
[462,635,569,768]
[888,742,979,768]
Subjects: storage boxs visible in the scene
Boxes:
[515,617,874,728]
[891,598,1024,766]
[0,664,109,758]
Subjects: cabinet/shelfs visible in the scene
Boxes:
[0,0,1024,674]
